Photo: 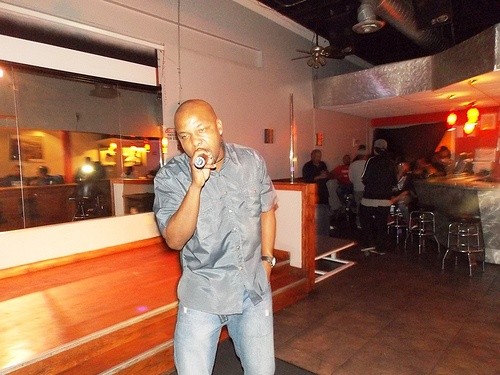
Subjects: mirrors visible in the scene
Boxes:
[0,59,166,232]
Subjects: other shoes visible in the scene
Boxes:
[370,249,386,255]
[360,245,376,252]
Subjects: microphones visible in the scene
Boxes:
[194,153,209,168]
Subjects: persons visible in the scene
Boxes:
[302,150,332,209]
[155,99,279,375]
[127,166,133,175]
[5,165,24,186]
[36,166,48,177]
[76,157,106,180]
[361,139,393,256]
[329,143,473,231]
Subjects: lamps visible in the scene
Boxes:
[306,58,327,69]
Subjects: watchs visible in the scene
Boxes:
[261,256,276,268]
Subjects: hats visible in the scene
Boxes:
[374,139,388,151]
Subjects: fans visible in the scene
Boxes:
[290,49,336,62]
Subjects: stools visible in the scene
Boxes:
[387,203,441,255]
[68,195,92,221]
[441,214,487,277]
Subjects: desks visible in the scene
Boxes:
[0,183,80,232]
[110,176,155,217]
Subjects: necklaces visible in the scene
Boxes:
[215,156,225,164]
